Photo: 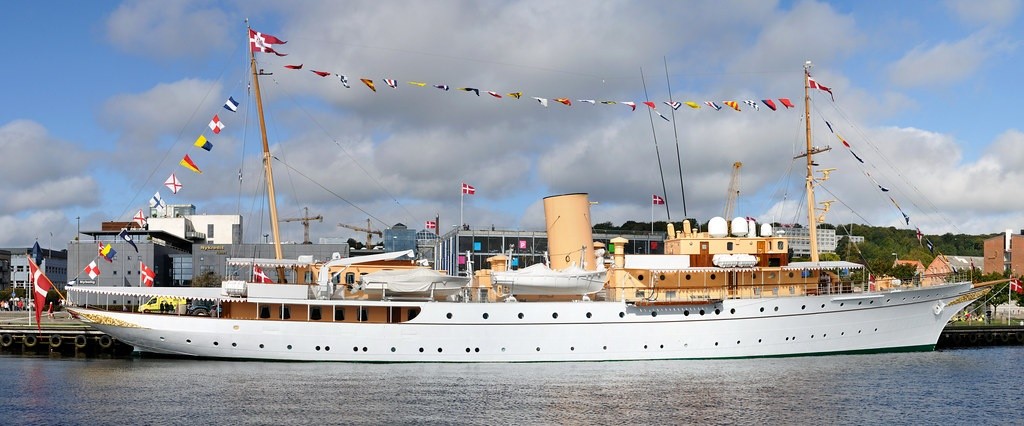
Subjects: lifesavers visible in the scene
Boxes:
[0,334,13,347]
[24,334,37,347]
[985,333,993,342]
[952,333,962,343]
[74,335,87,348]
[1015,332,1024,342]
[50,334,62,348]
[969,333,977,343]
[99,335,112,348]
[1001,333,1008,343]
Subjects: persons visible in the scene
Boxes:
[210,306,217,317]
[160,301,175,313]
[0,298,24,312]
[48,302,55,319]
[964,308,991,323]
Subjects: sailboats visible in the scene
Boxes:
[58,20,1019,364]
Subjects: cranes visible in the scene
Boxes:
[338,218,381,249]
[278,207,323,244]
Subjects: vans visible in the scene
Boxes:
[138,296,186,313]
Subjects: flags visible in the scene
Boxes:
[653,195,665,205]
[134,210,148,227]
[98,242,118,263]
[180,154,203,175]
[165,174,183,194]
[150,193,167,213]
[807,72,834,101]
[248,28,288,58]
[223,96,239,112]
[1010,276,1024,295]
[118,230,139,253]
[426,221,436,229]
[826,122,957,275]
[140,262,157,287]
[462,183,475,195]
[194,135,213,151]
[28,256,54,340]
[285,65,795,122]
[84,261,101,280]
[253,264,273,283]
[208,115,226,134]
[745,217,755,222]
